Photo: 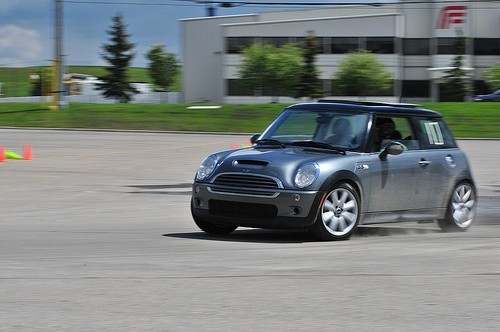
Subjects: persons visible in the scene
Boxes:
[352,117,408,155]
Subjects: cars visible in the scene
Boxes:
[473,88,500,102]
[191,100,477,241]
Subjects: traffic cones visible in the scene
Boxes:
[23,144,33,160]
[0,145,7,162]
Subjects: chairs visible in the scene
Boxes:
[326,117,414,147]
